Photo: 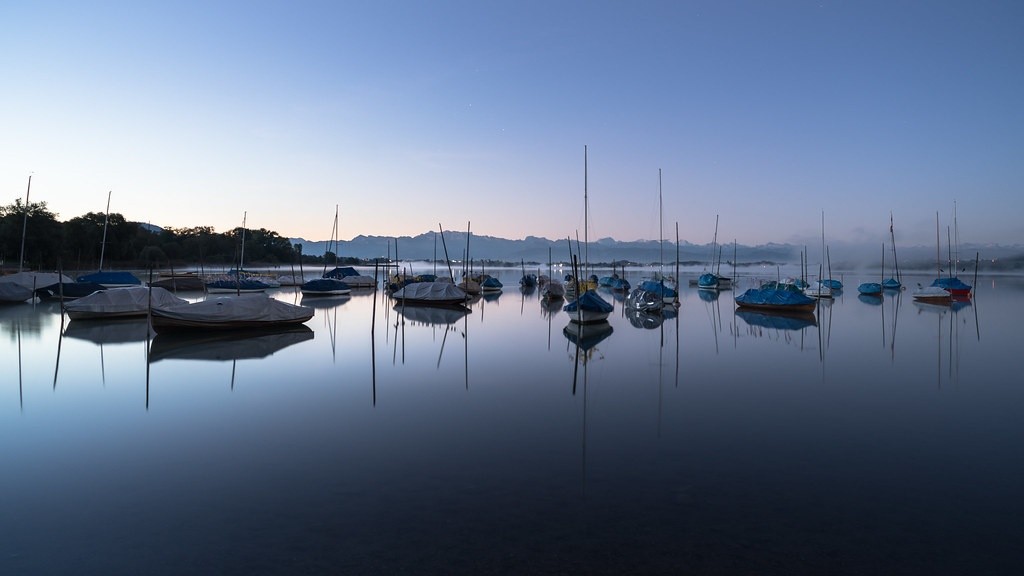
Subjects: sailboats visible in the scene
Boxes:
[910,211,973,299]
[320,203,380,287]
[882,286,902,355]
[561,321,614,471]
[532,143,680,322]
[857,241,885,294]
[911,297,975,385]
[857,293,886,348]
[880,211,903,289]
[686,214,737,290]
[698,288,723,355]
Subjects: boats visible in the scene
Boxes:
[145,321,315,411]
[733,209,844,312]
[0,174,380,295]
[57,284,190,320]
[143,258,315,338]
[299,276,351,296]
[517,257,537,286]
[519,285,535,316]
[392,301,473,368]
[298,294,353,365]
[60,320,160,384]
[808,296,835,350]
[832,289,844,299]
[383,219,503,305]
[736,306,820,354]
